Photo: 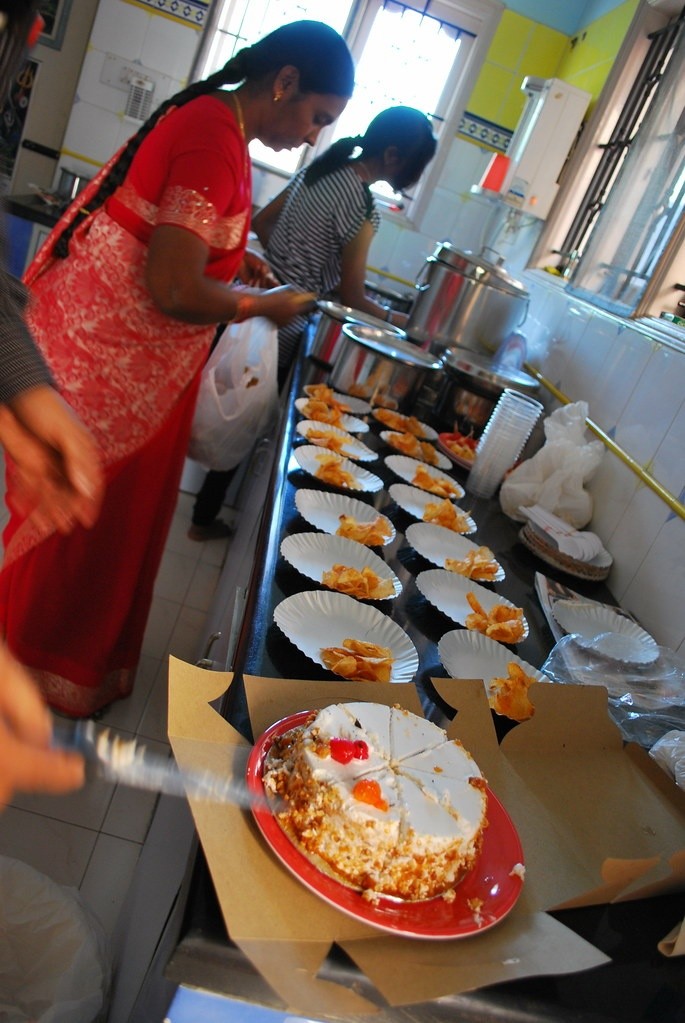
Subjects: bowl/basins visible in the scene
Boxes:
[58,166,92,202]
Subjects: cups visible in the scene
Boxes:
[464,389,543,500]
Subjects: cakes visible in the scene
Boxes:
[266,701,487,901]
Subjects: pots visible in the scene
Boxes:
[331,321,442,415]
[436,346,541,444]
[302,298,408,372]
[407,238,530,362]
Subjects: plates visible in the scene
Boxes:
[243,706,525,942]
[435,431,526,482]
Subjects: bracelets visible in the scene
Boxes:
[384,305,392,323]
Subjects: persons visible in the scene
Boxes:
[187,105,435,542]
[0,240,105,814]
[0,19,355,719]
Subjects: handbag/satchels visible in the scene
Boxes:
[189,312,281,472]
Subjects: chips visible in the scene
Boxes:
[303,381,539,720]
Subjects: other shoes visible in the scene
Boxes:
[187,518,233,541]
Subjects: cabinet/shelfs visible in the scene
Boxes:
[108,368,295,1023]
